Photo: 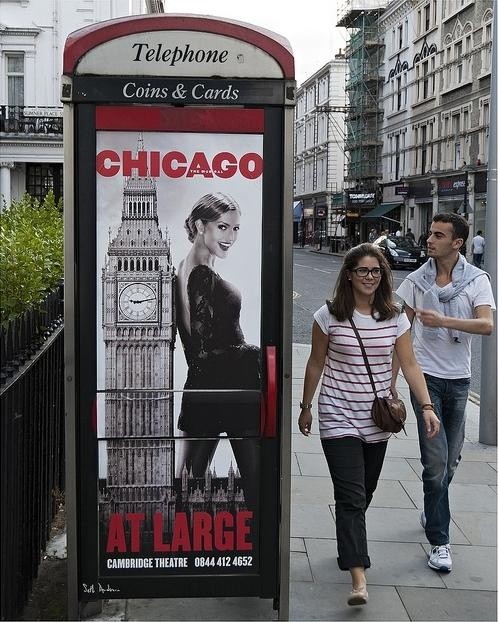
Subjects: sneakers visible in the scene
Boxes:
[419,511,426,528]
[428,545,452,571]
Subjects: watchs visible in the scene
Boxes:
[300,401,313,409]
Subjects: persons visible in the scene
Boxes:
[300,232,306,246]
[297,242,441,606]
[369,228,429,248]
[391,213,497,572]
[314,228,321,238]
[469,230,485,269]
[173,192,261,513]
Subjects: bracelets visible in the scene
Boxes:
[422,403,435,412]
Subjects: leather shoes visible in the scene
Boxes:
[348,589,368,605]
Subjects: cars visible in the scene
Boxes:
[371,234,429,270]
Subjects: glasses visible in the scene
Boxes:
[350,267,383,278]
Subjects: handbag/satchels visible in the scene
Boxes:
[372,397,406,433]
[384,246,394,263]
